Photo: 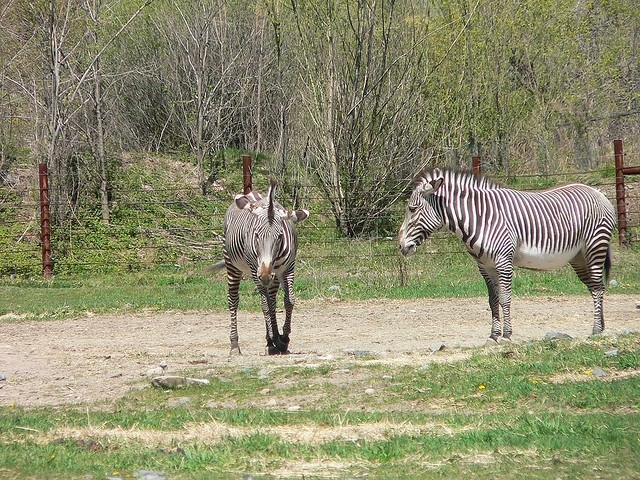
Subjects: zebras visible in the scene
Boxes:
[397,166,615,347]
[223,177,309,356]
